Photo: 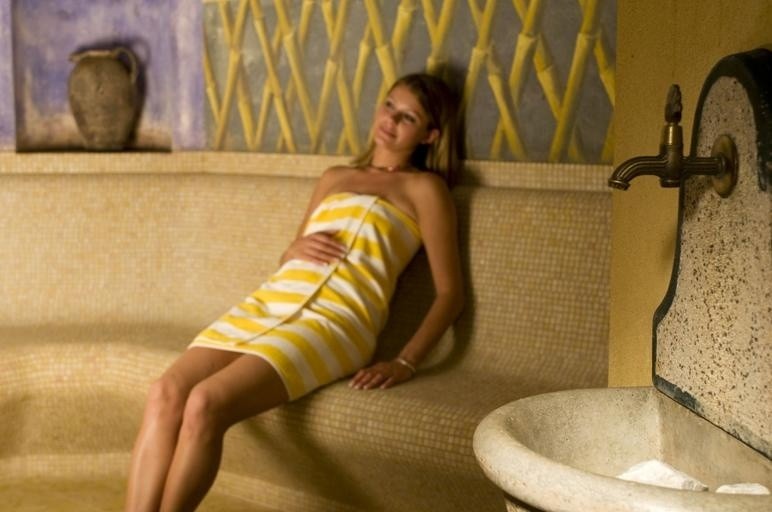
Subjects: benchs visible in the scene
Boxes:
[0,150,613,512]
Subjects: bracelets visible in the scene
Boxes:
[395,357,418,374]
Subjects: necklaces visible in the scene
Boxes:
[367,162,411,174]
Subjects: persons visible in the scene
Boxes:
[123,72,466,511]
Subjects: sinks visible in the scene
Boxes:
[502,382,771,496]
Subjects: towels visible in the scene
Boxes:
[188,189,423,403]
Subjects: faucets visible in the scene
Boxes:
[603,82,740,198]
